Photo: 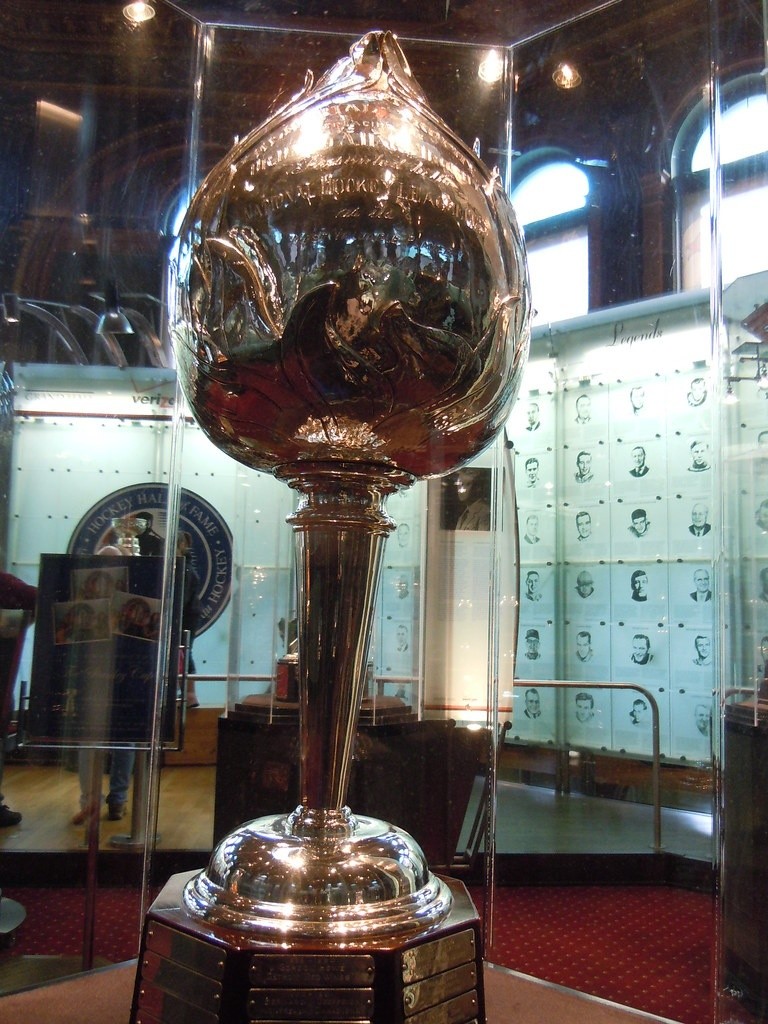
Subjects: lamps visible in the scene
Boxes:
[122,0,156,23]
[94,280,134,336]
[551,62,582,88]
[478,49,507,82]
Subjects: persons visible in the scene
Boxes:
[524,688,541,719]
[575,511,593,542]
[630,387,646,415]
[688,440,711,471]
[575,631,594,662]
[629,699,647,724]
[391,521,413,704]
[627,509,651,537]
[630,570,648,602]
[75,742,134,823]
[525,458,539,488]
[689,503,711,536]
[631,635,653,664]
[575,395,591,424]
[687,377,707,407]
[629,446,650,478]
[525,403,540,431]
[455,469,490,531]
[691,568,714,737]
[575,451,593,483]
[755,373,768,679]
[524,571,542,601]
[56,572,160,642]
[576,693,595,722]
[524,629,541,660]
[168,532,200,709]
[523,515,540,544]
[574,571,594,599]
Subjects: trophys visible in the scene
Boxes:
[125,31,532,1024]
[111,516,147,556]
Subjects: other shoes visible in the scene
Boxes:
[109,802,127,819]
[72,796,104,825]
[0,805,22,828]
